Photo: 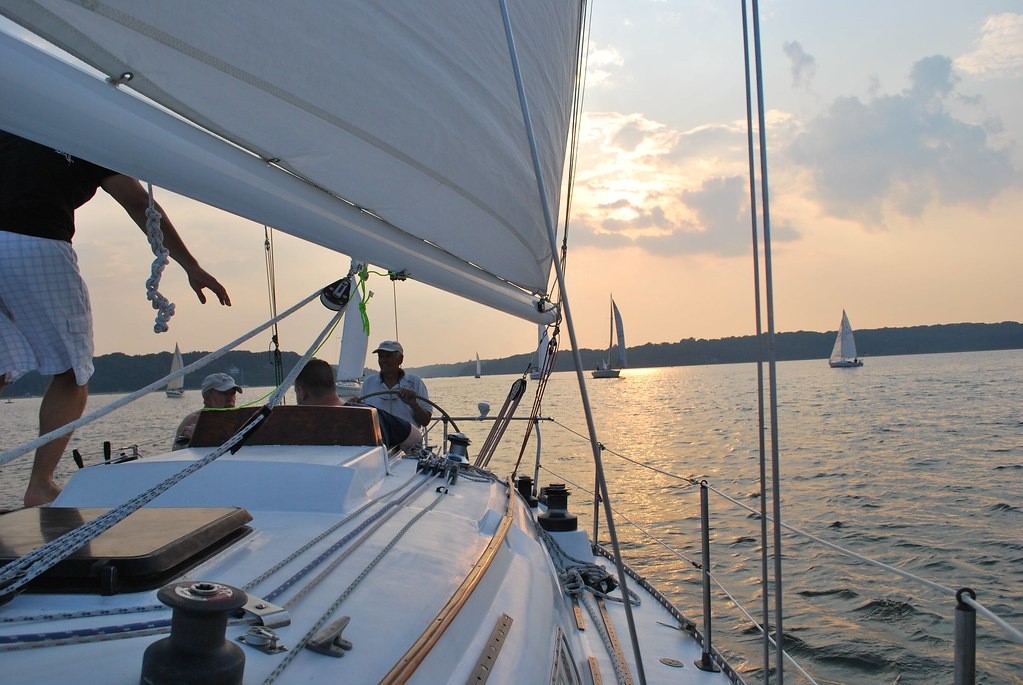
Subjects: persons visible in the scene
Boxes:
[294,359,422,450]
[0,127,232,507]
[362,340,432,429]
[172,373,242,451]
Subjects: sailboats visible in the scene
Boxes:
[591,292,628,378]
[828,308,864,368]
[474,352,482,379]
[165,341,186,398]
[335,276,369,398]
[529,321,550,380]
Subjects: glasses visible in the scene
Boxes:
[378,380,402,401]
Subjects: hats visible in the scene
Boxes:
[372,340,403,356]
[202,373,243,397]
